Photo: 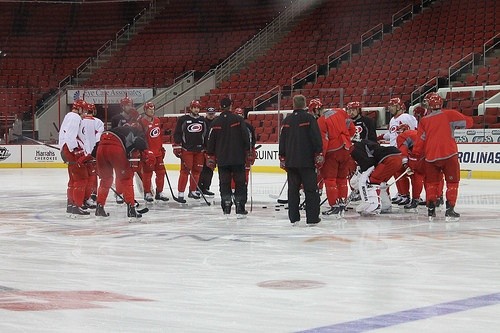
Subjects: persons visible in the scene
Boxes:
[58,97,256,222]
[279,91,473,226]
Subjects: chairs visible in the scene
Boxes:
[0,0,500,136]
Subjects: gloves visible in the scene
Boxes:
[78,154,93,167]
[313,152,325,169]
[142,149,156,167]
[204,153,217,170]
[278,156,287,172]
[171,144,183,158]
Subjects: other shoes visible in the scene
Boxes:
[290,217,321,226]
[349,190,362,204]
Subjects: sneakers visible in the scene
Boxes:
[144,192,169,202]
[198,187,215,198]
[66,195,109,219]
[127,204,142,222]
[220,199,248,219]
[322,198,346,220]
[178,191,200,199]
[391,195,460,222]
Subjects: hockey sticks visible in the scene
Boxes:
[320,198,327,206]
[246,126,253,211]
[53,123,59,132]
[84,163,149,214]
[269,179,287,199]
[94,171,139,207]
[162,161,186,203]
[255,145,261,149]
[345,189,359,206]
[299,133,356,210]
[12,133,60,150]
[181,157,210,206]
[387,169,412,187]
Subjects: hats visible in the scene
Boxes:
[206,107,215,119]
[220,98,232,108]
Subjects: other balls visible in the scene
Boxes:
[275,206,280,207]
[275,209,280,211]
[262,207,267,208]
[280,205,284,206]
[285,208,289,209]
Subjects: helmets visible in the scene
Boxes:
[346,101,362,109]
[388,98,406,109]
[190,100,201,108]
[143,101,155,117]
[424,92,443,108]
[307,98,324,112]
[413,107,428,116]
[121,97,134,106]
[234,108,242,114]
[72,99,97,115]
